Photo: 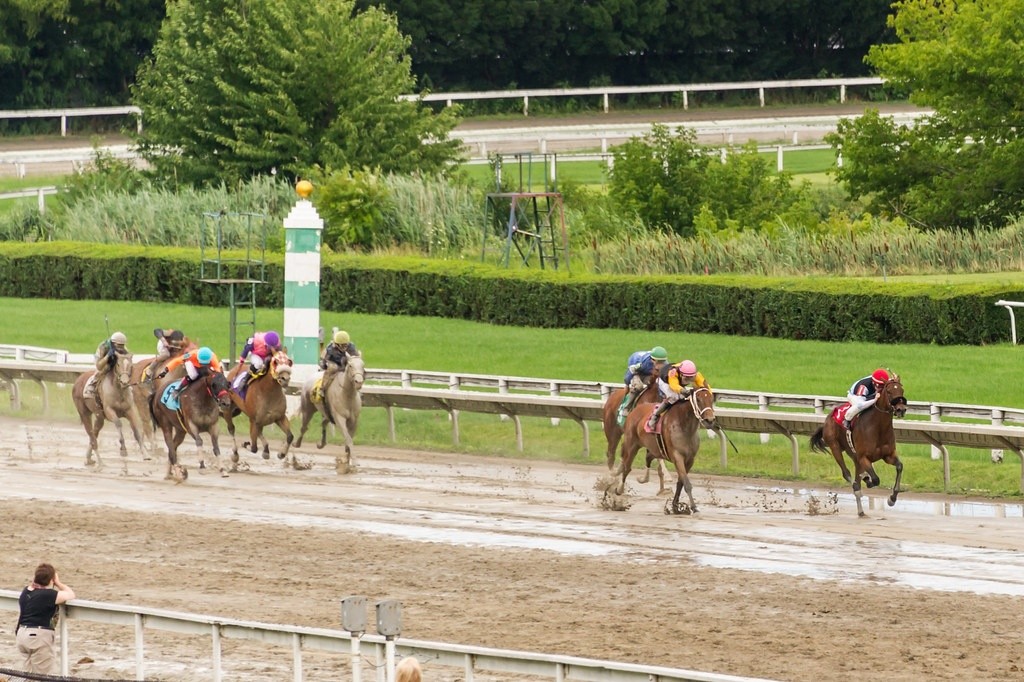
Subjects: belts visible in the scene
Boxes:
[21,625,53,630]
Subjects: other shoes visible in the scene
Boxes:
[241,377,247,388]
[144,365,153,376]
[617,408,625,424]
[842,419,850,429]
[649,413,660,430]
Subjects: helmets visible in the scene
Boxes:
[335,331,350,344]
[649,346,666,360]
[111,332,127,346]
[872,369,889,385]
[680,360,697,377]
[197,346,211,365]
[264,330,279,346]
[171,331,183,344]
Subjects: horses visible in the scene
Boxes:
[610,379,715,515]
[601,359,674,495]
[221,346,293,462]
[292,351,366,469]
[72,352,186,465]
[809,374,907,517]
[142,356,230,484]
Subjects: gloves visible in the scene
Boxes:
[681,390,690,397]
[108,348,115,357]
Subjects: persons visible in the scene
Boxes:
[145,330,184,377]
[844,369,888,429]
[647,359,714,430]
[96,333,130,372]
[622,346,668,417]
[318,331,360,397]
[156,347,222,398]
[14,564,75,682]
[236,331,279,391]
